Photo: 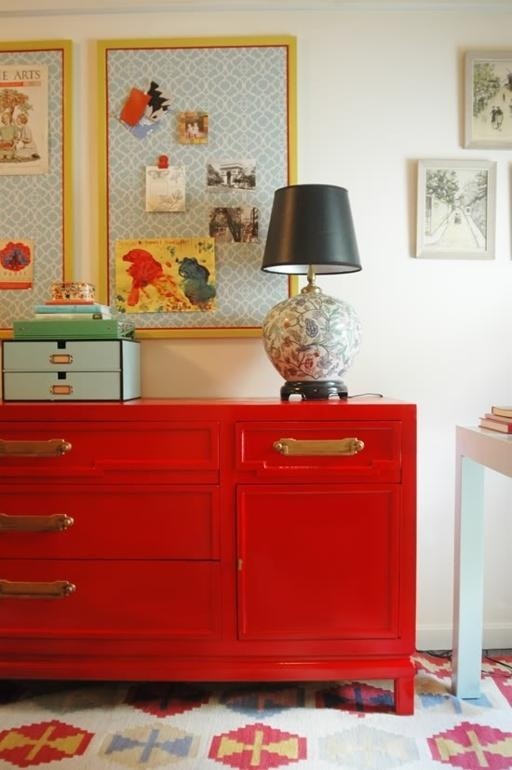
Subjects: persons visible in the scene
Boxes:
[186,123,193,138]
[191,121,200,138]
[13,112,42,161]
[1,107,20,160]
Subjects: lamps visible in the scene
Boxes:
[260,180,365,402]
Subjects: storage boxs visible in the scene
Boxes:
[1,341,141,401]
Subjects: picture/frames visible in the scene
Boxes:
[1,34,299,336]
[414,43,512,260]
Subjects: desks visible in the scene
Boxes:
[454,425,512,702]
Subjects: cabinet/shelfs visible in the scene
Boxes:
[2,404,224,679]
[227,405,414,712]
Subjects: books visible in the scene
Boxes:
[33,312,112,320]
[491,405,511,418]
[485,412,512,424]
[45,300,93,304]
[31,304,111,313]
[477,417,512,434]
